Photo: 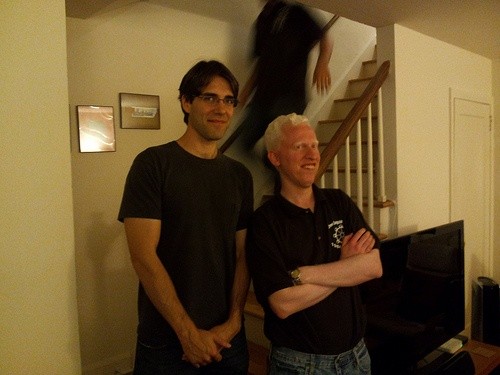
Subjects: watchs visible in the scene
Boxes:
[290,268,302,285]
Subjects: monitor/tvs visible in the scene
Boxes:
[364,219,465,375]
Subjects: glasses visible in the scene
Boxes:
[185,94,237,106]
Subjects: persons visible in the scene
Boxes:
[118,60,254,375]
[238,0,333,196]
[244,114,388,375]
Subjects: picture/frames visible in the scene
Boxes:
[77,104,116,153]
[119,93,160,130]
